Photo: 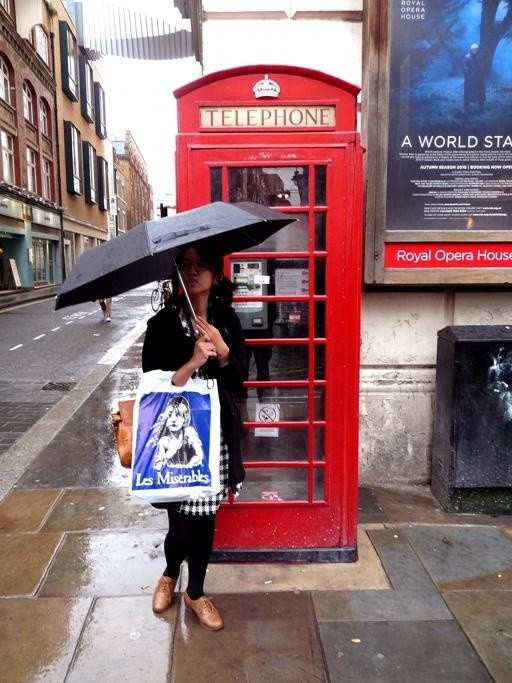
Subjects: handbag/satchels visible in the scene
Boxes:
[112,399,135,468]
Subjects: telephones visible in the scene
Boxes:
[232,282,253,292]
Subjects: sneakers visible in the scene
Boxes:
[104,316,112,322]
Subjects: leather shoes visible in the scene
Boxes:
[183,587,224,631]
[153,575,178,613]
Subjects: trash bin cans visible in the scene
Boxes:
[431,324,511,511]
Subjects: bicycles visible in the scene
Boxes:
[150,279,174,312]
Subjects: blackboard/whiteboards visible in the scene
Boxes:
[9,258,22,288]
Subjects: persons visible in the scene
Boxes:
[141,239,250,632]
[238,335,274,441]
[98,296,112,322]
[147,395,205,471]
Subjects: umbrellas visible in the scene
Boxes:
[52,201,296,335]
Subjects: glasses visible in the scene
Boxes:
[179,259,210,272]
